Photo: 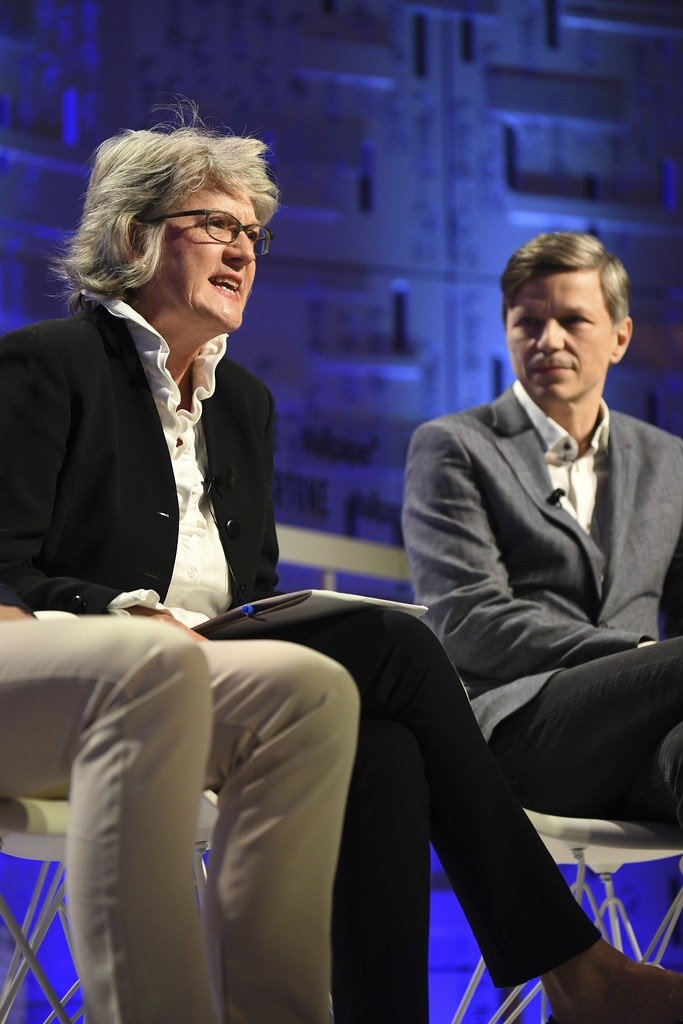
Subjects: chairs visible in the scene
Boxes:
[2,790,227,1024]
[436,810,683,1024]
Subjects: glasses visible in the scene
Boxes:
[145,209,274,257]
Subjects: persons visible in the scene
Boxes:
[401,232,681,846]
[0,618,360,1023]
[0,102,683,1024]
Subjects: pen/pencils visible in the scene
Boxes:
[239,606,268,623]
